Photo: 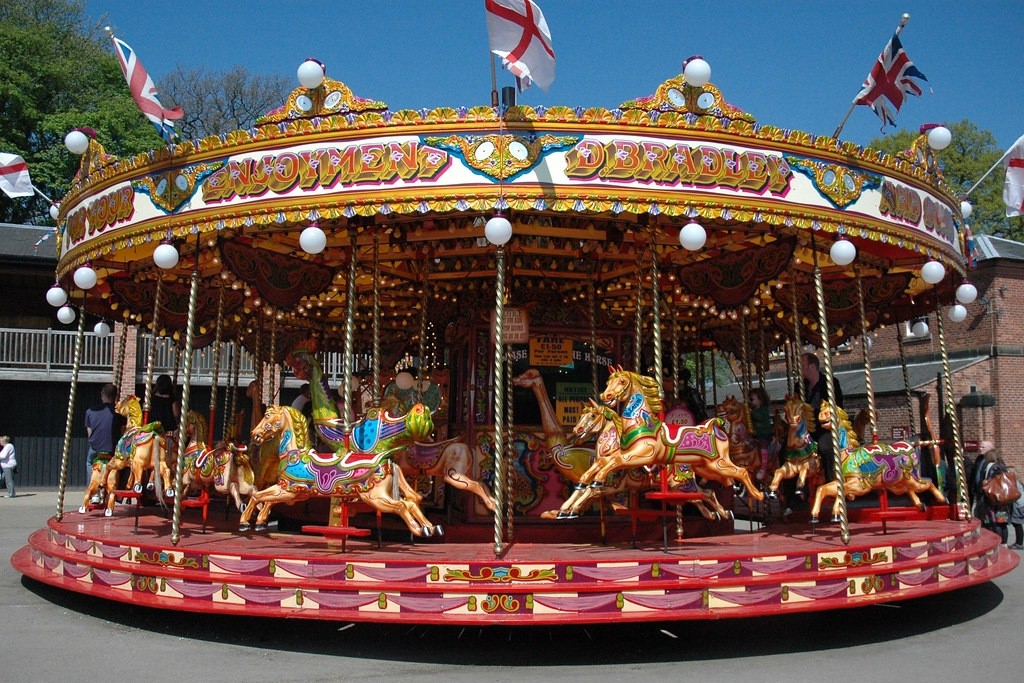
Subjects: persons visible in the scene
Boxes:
[147,374,180,434]
[945,440,1024,550]
[0,436,17,497]
[778,352,844,510]
[85,384,129,505]
[749,387,773,441]
[291,383,316,431]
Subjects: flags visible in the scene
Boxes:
[0,153,35,199]
[113,37,184,146]
[852,33,933,134]
[1002,136,1024,218]
[484,0,556,93]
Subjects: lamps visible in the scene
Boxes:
[57,303,76,324]
[913,317,928,336]
[949,300,967,321]
[297,58,326,89]
[961,197,972,218]
[682,55,711,87]
[830,233,856,266]
[679,217,707,249]
[153,237,179,270]
[413,380,431,392]
[397,368,414,390]
[956,276,977,304]
[64,128,95,154]
[921,123,952,150]
[94,318,111,338]
[299,220,326,253]
[485,210,513,245]
[46,282,67,306]
[921,257,946,283]
[73,262,96,289]
[339,375,359,396]
[50,200,61,219]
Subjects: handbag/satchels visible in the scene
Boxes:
[982,465,1021,506]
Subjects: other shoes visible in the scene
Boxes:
[4,494,15,498]
[1008,543,1023,550]
[1001,543,1008,549]
[91,496,101,503]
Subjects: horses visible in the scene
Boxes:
[170,408,271,515]
[766,397,856,502]
[79,452,118,518]
[390,436,506,513]
[809,398,950,524]
[97,394,174,500]
[557,362,768,519]
[238,404,444,538]
[715,395,782,498]
